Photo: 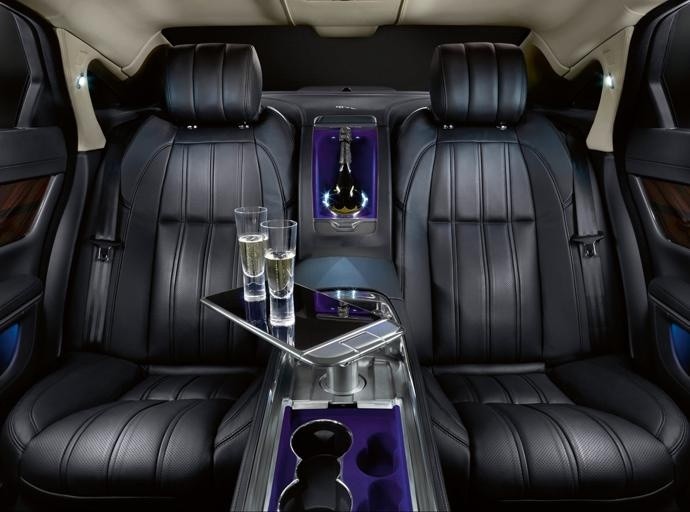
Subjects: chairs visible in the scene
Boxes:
[0,43,296,512]
[391,39,689,511]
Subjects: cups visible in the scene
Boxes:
[260,217,297,327]
[234,205,268,303]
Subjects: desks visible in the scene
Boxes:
[198,279,408,395]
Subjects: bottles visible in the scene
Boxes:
[322,126,369,219]
